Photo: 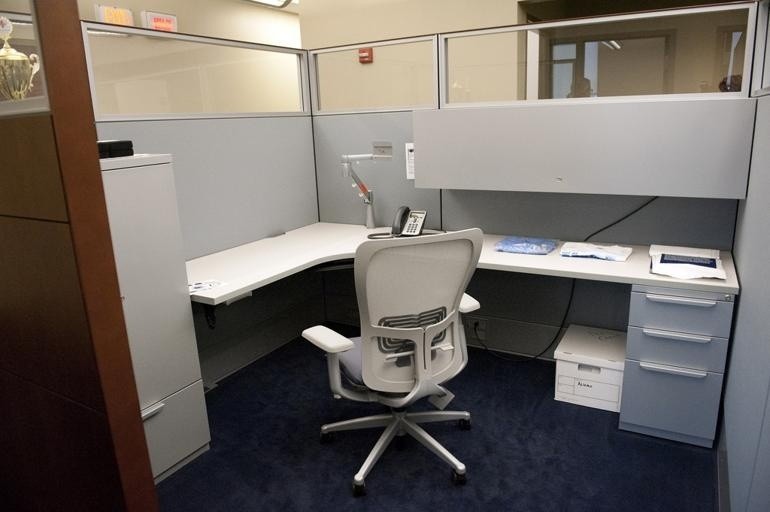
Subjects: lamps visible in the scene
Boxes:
[340,140,394,228]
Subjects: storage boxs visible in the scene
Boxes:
[553,324,627,413]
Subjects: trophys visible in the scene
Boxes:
[0,14,41,101]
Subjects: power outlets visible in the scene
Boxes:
[465,315,487,341]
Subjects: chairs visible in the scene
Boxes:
[301,227,484,495]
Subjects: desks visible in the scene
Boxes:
[185,222,742,451]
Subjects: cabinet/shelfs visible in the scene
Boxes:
[413,96,756,201]
[101,153,212,484]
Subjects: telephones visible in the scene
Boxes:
[392,206,427,236]
